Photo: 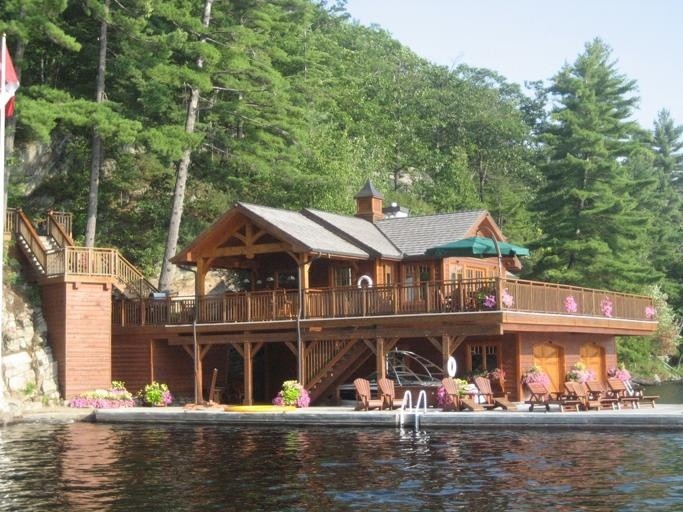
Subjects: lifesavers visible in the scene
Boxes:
[357,275,372,289]
[447,357,457,377]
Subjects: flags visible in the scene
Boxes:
[0,33,21,120]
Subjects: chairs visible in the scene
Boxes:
[352,377,404,411]
[524,378,660,413]
[442,376,518,412]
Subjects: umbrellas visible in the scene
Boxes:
[423,225,531,277]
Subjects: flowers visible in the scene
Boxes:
[272,380,309,408]
[563,294,613,318]
[483,286,515,308]
[644,304,658,319]
[135,380,172,406]
[519,362,631,386]
